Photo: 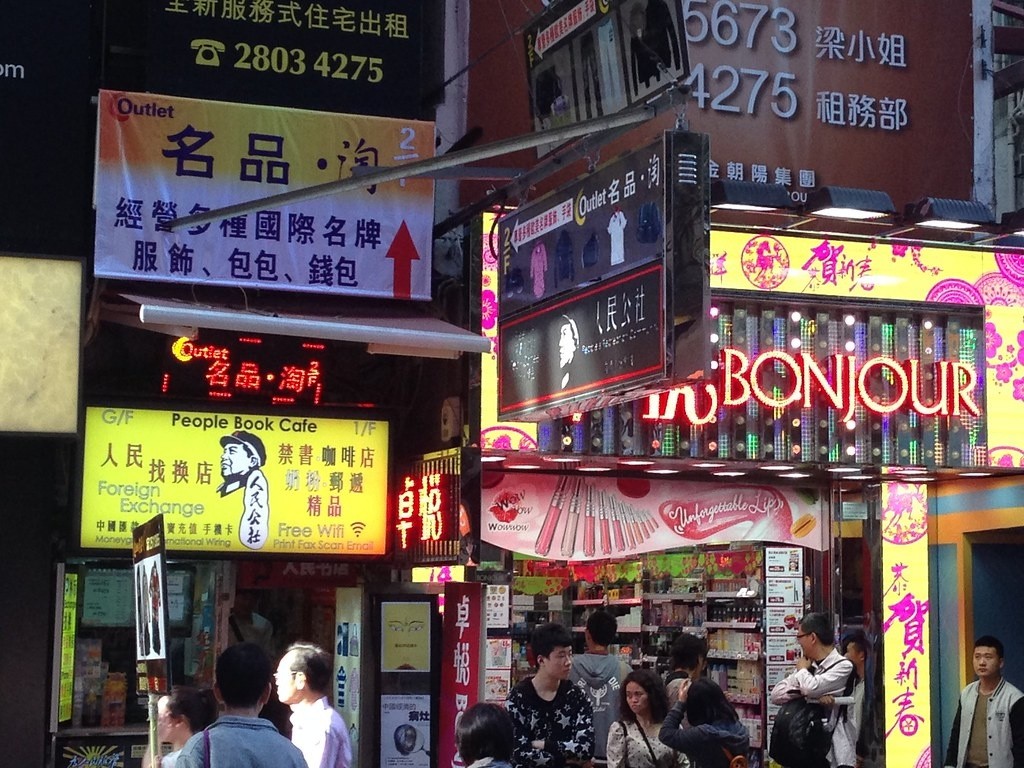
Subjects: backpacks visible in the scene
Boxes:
[767,660,857,767]
[722,747,752,768]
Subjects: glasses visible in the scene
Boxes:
[274,671,298,679]
[797,633,812,638]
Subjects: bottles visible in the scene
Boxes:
[713,608,761,623]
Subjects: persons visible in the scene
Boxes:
[771,612,857,768]
[504,623,597,768]
[273,642,351,768]
[944,635,1024,768]
[662,630,708,730]
[843,637,865,743]
[139,686,220,768]
[606,668,690,768]
[568,612,634,768]
[658,676,750,768]
[174,641,308,768]
[455,703,514,768]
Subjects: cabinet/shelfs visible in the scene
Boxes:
[511,579,767,751]
